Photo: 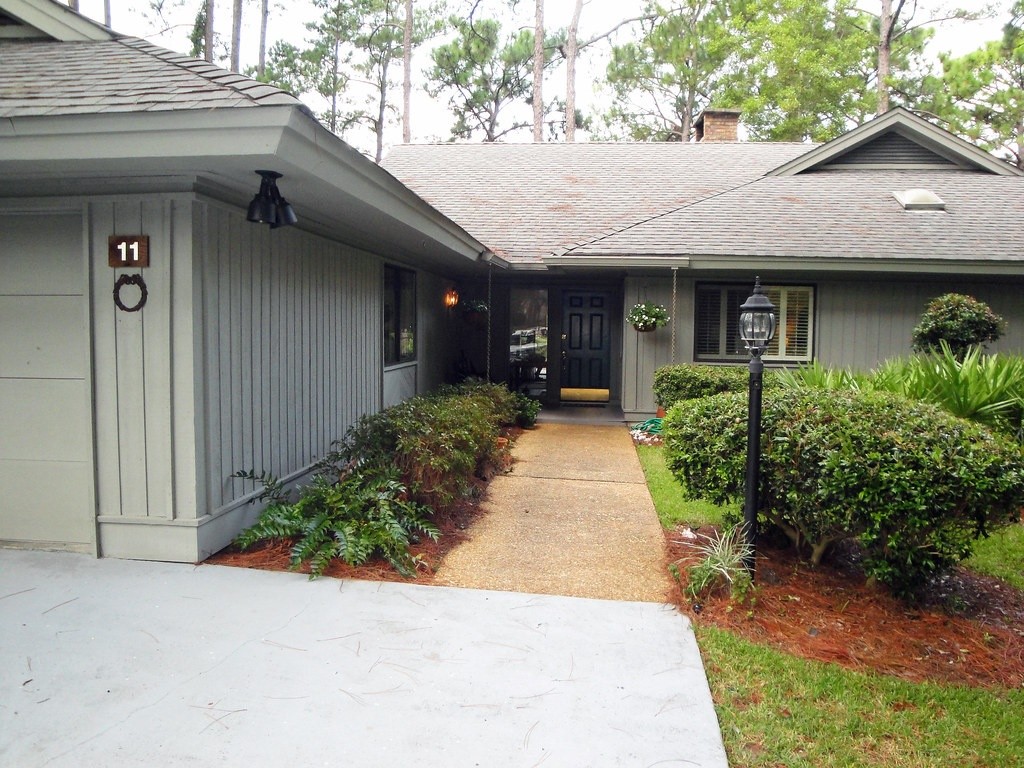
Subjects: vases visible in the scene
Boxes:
[633,324,655,332]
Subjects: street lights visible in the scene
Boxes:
[738,277,777,584]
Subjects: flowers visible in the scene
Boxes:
[625,303,671,330]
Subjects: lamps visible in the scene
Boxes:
[245,170,299,230]
[739,273,775,360]
